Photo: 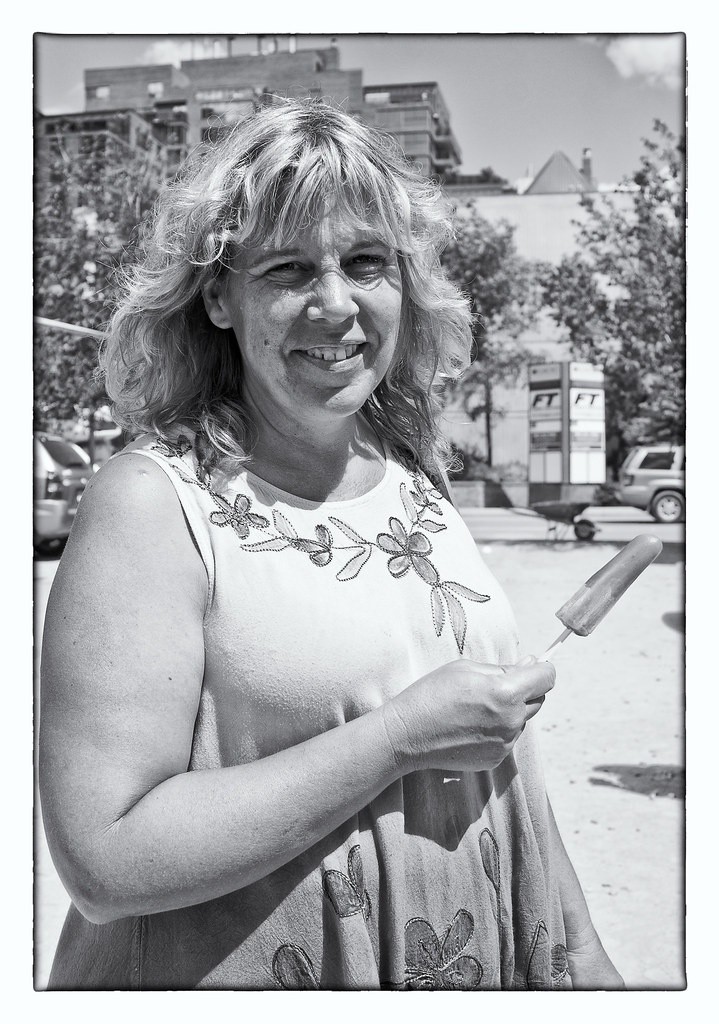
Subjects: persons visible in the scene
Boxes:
[37,96,631,991]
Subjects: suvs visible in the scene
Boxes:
[34,431,99,562]
[616,444,686,523]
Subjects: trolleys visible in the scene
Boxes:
[503,498,604,542]
